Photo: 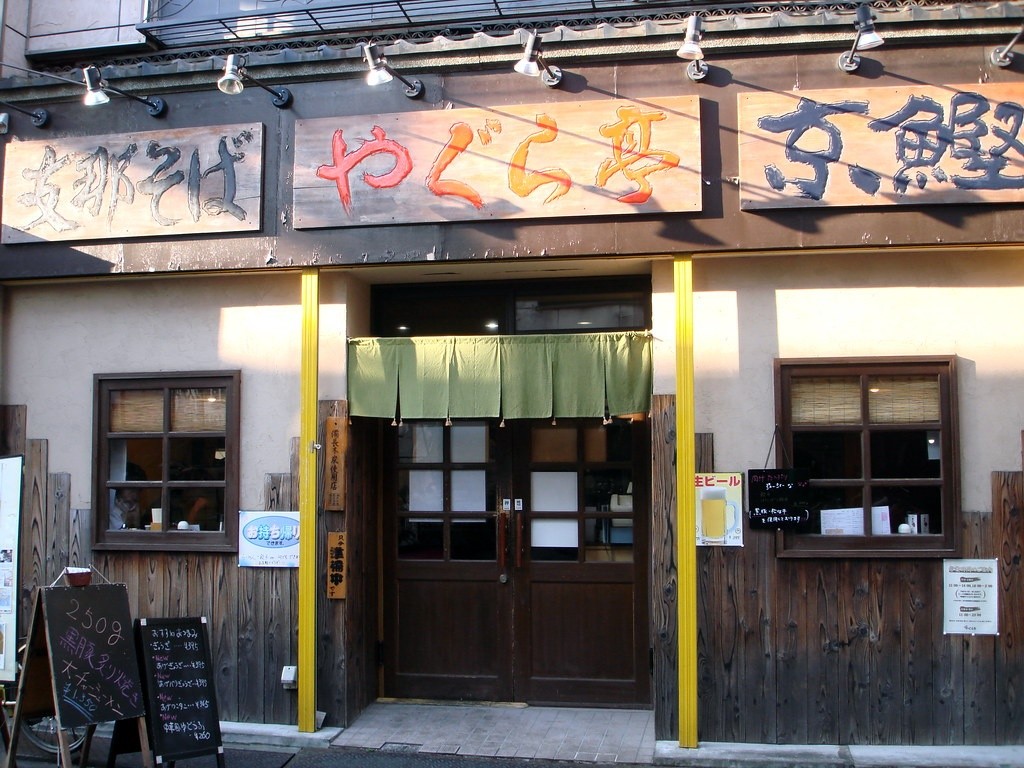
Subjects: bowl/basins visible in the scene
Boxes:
[63,571,92,587]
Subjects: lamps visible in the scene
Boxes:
[676,13,710,82]
[364,37,425,100]
[216,54,293,107]
[514,28,563,91]
[82,63,168,118]
[840,4,885,74]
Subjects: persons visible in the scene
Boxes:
[109,461,218,531]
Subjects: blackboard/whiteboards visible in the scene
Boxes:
[138,617,223,764]
[42,583,145,731]
[748,467,822,535]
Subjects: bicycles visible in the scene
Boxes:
[0,634,97,755]
